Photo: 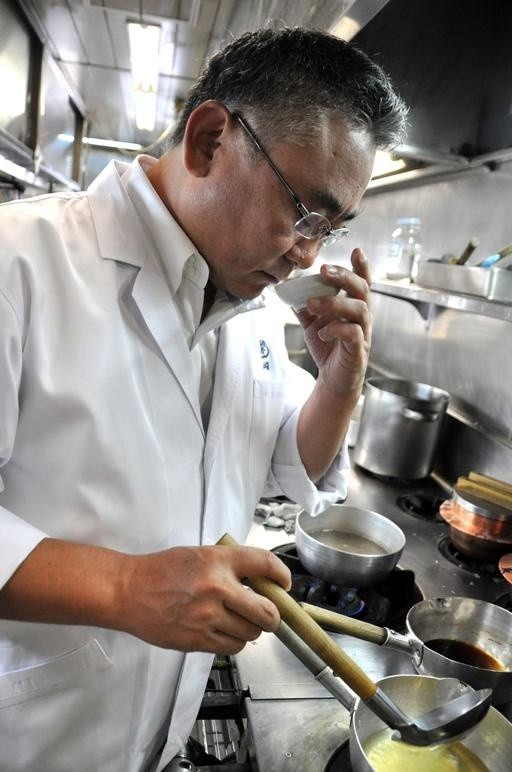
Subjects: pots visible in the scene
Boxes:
[271,621,511,772]
[430,471,509,561]
[355,375,449,478]
[296,502,407,587]
[300,592,511,703]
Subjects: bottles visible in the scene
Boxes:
[385,218,421,281]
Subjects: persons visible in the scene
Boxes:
[0,25,413,772]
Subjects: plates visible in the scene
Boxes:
[275,272,343,313]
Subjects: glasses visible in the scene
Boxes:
[228,107,352,249]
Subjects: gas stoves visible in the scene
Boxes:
[235,446,510,772]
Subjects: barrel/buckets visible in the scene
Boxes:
[352,376,452,481]
